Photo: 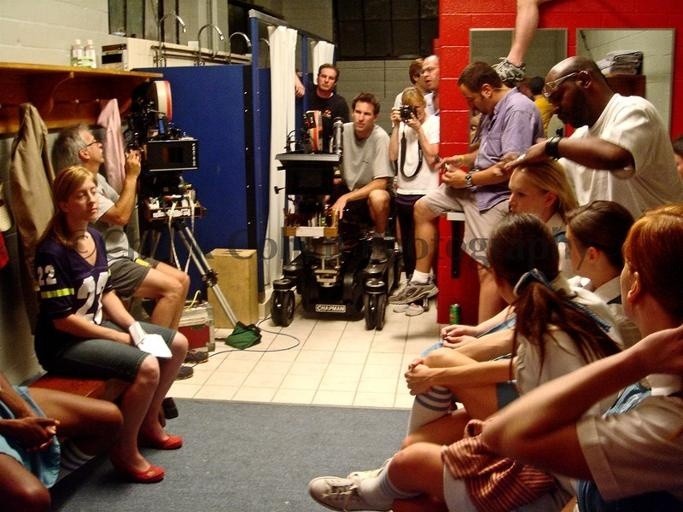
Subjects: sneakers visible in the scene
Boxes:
[491,57,528,82]
[175,366,193,380]
[309,476,394,512]
[183,352,208,363]
[388,279,439,304]
[393,303,409,313]
[370,236,386,265]
[405,303,425,316]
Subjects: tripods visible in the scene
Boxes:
[131,222,252,347]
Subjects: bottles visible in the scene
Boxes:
[85,39,97,68]
[71,38,85,68]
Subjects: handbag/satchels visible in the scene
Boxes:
[224,321,262,350]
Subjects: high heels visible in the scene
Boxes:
[109,456,164,483]
[137,430,182,449]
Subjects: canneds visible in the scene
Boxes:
[449,304,461,325]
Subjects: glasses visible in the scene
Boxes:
[541,71,578,98]
[420,66,437,74]
[87,134,102,146]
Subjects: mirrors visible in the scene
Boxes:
[577,27,675,140]
[469,27,568,151]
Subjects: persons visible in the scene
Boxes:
[296,0,681,434]
[25,166,182,484]
[0,369,125,511]
[52,124,190,331]
[483,204,683,512]
[402,214,624,445]
[308,203,682,511]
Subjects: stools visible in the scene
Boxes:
[24,372,130,433]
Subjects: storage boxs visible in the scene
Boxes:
[207,248,260,328]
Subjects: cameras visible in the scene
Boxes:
[399,104,417,124]
